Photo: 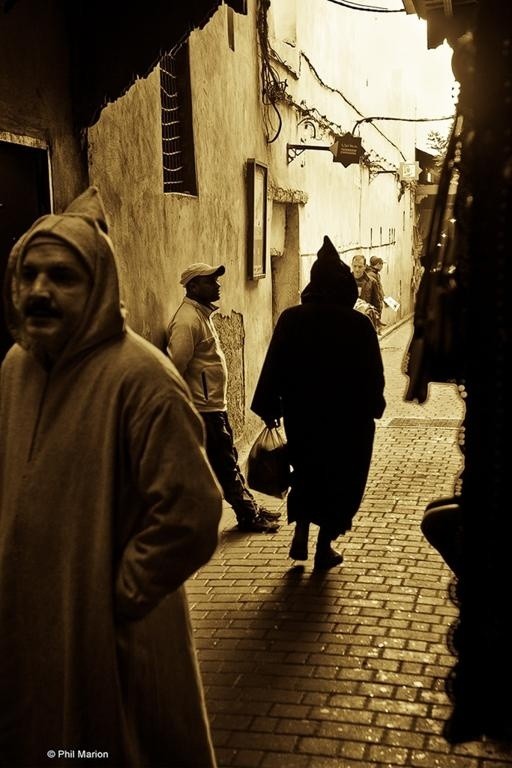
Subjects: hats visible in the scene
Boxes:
[179,263,226,288]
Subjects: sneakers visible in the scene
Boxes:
[315,551,343,570]
[289,541,309,561]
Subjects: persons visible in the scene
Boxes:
[167,259,282,531]
[248,232,388,569]
[349,254,381,323]
[0,186,223,768]
[369,257,386,309]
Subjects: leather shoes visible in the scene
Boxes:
[258,509,281,522]
[236,516,280,532]
